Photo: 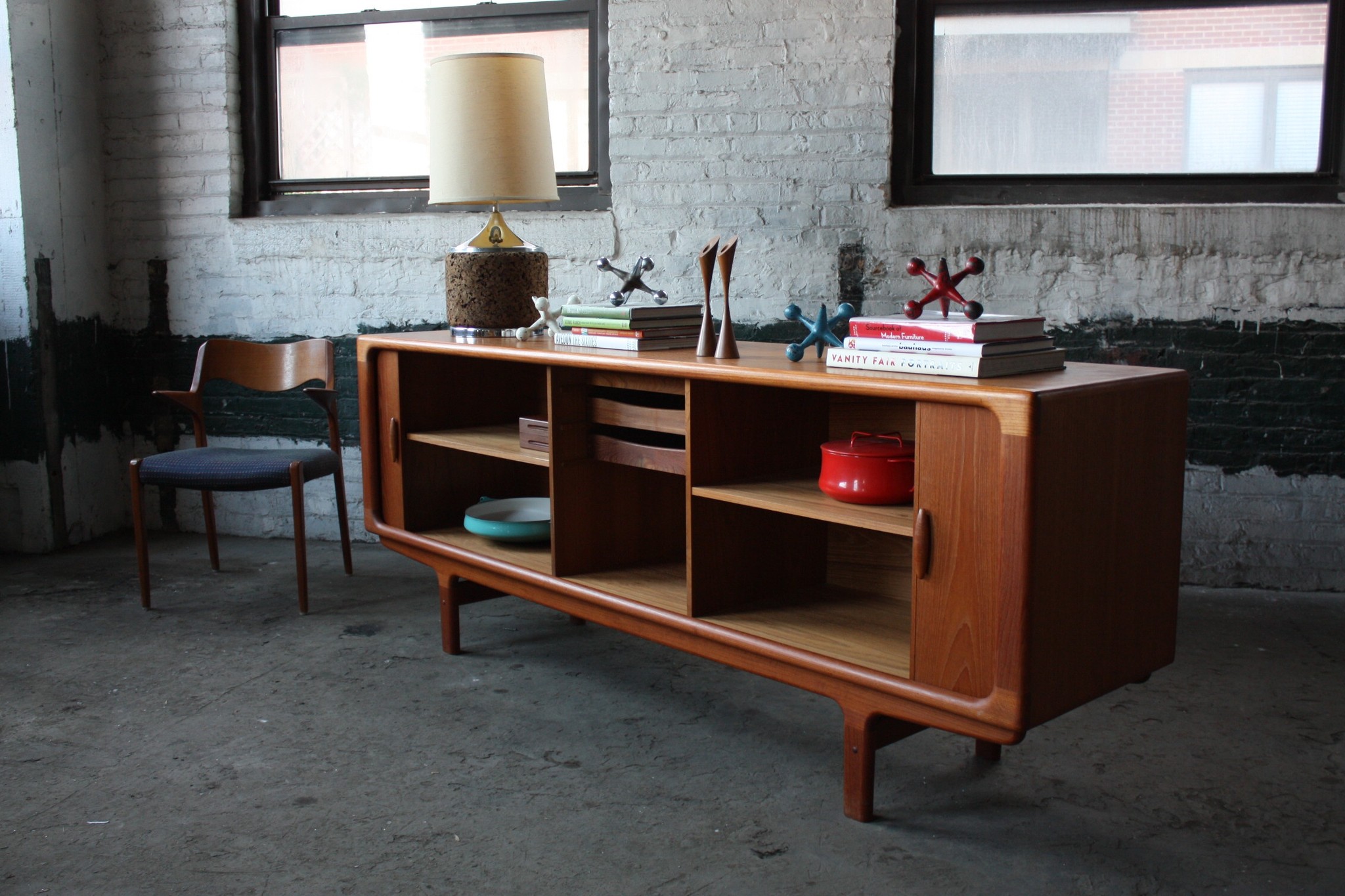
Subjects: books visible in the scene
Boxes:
[554,302,720,351]
[825,310,1067,378]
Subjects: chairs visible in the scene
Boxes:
[129,338,352,616]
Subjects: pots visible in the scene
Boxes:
[817,430,915,506]
[464,496,552,543]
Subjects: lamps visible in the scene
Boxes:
[427,52,561,337]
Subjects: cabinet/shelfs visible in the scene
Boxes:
[356,320,1187,822]
